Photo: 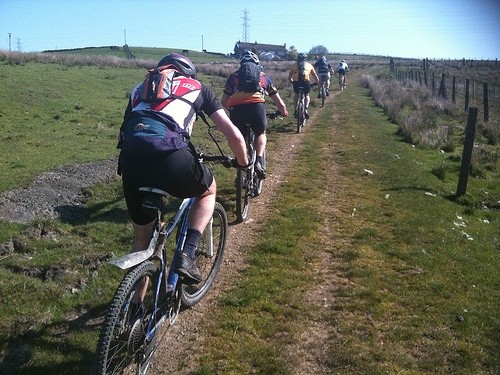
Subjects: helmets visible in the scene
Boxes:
[298,53,307,58]
[321,56,326,60]
[158,53,196,79]
[240,51,259,65]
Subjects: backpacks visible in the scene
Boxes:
[238,62,260,92]
[138,64,183,101]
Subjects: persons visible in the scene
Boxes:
[289,53,350,119]
[222,51,287,181]
[118,53,253,315]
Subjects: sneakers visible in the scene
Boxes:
[175,251,202,285]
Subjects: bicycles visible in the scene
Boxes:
[317,73,333,107]
[234,110,283,222]
[292,81,319,133]
[95,150,257,375]
[337,70,348,91]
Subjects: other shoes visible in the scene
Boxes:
[305,113,309,119]
[254,161,267,179]
